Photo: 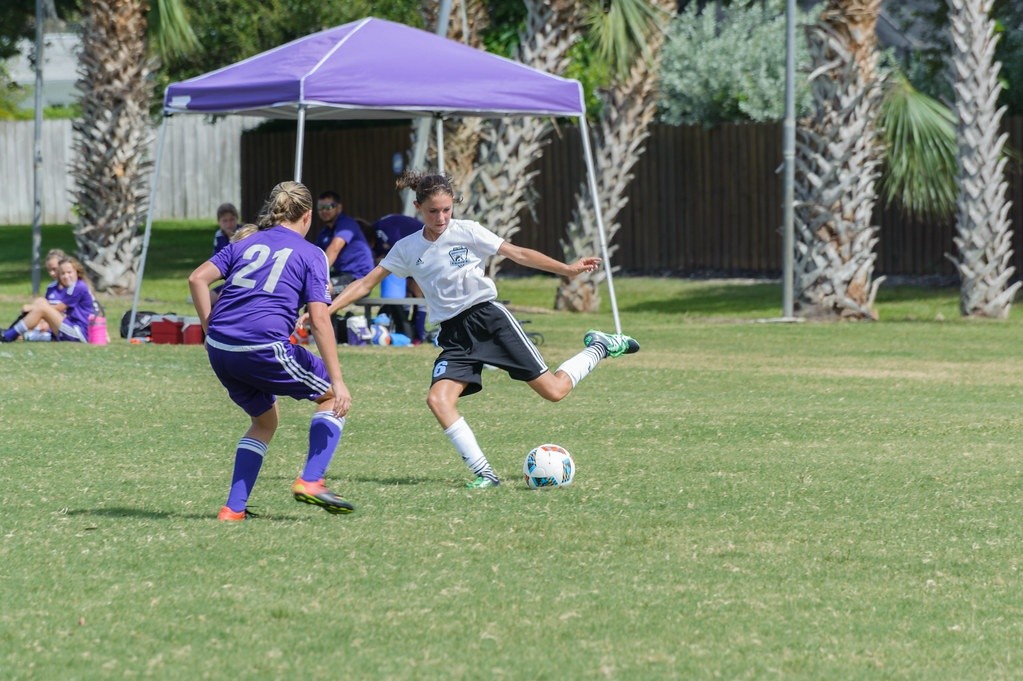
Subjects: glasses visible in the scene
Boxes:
[317,203,337,210]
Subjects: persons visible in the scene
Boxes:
[210,204,244,308]
[298,171,641,489]
[188,180,355,521]
[358,215,429,346]
[0,249,105,344]
[313,190,375,301]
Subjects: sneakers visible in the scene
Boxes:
[465,477,499,489]
[584,330,639,358]
[292,476,354,515]
[216,505,259,521]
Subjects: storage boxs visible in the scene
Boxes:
[181,317,204,345]
[150,314,184,345]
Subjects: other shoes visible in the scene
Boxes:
[0,329,9,343]
[23,331,52,342]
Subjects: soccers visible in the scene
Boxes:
[522,444,576,489]
[370,325,391,346]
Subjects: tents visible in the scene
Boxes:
[124,16,624,342]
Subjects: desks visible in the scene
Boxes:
[355,297,431,325]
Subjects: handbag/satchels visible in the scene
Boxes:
[88,300,106,344]
[120,309,177,338]
